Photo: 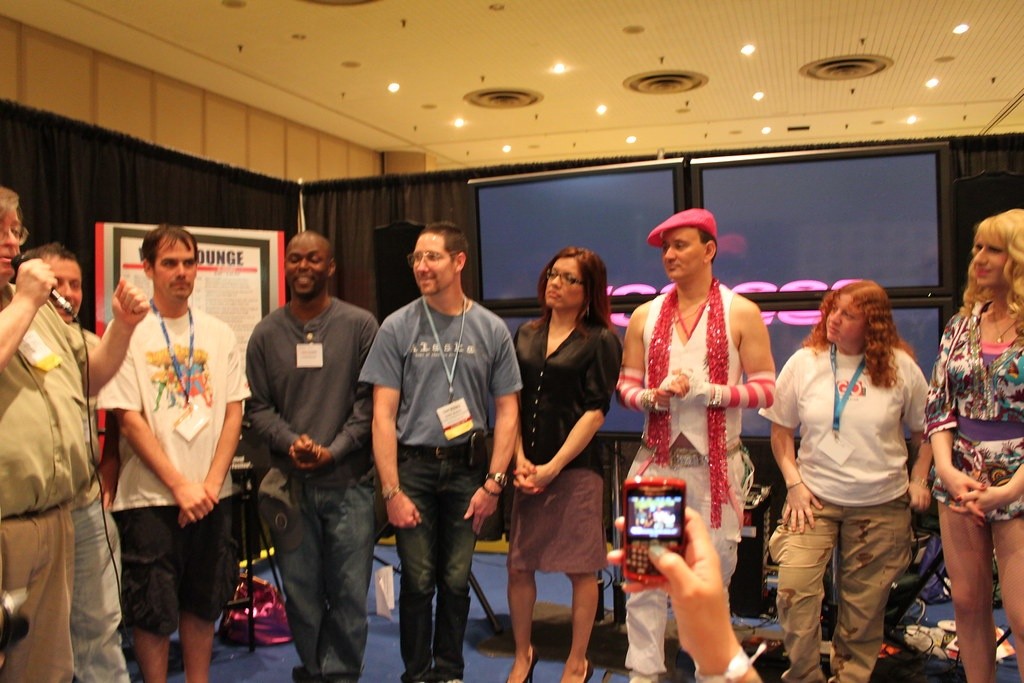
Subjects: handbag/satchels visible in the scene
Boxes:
[219,579,293,644]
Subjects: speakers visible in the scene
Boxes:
[948,167,1024,307]
[374,221,428,324]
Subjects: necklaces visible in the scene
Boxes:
[674,301,709,339]
[991,302,1016,343]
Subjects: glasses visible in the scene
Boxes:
[0,222,29,245]
[545,267,585,287]
[407,250,461,269]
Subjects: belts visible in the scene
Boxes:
[397,442,486,461]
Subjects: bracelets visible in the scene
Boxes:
[485,472,507,487]
[909,477,927,487]
[483,486,502,497]
[787,480,804,490]
[695,651,749,683]
[384,485,401,503]
[640,388,654,411]
[710,384,721,408]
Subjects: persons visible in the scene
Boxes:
[924,208,1024,683]
[616,208,775,683]
[22,242,130,683]
[0,187,150,683]
[358,218,523,682]
[96,224,252,683]
[758,279,934,683]
[244,230,380,682]
[508,244,623,683]
[616,505,764,683]
[631,496,676,529]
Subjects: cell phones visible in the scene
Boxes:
[621,477,685,588]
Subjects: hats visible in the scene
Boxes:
[646,207,717,248]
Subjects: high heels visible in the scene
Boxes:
[559,657,593,683]
[505,644,539,683]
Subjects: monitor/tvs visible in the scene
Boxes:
[472,140,959,443]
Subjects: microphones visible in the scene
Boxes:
[9,253,77,319]
